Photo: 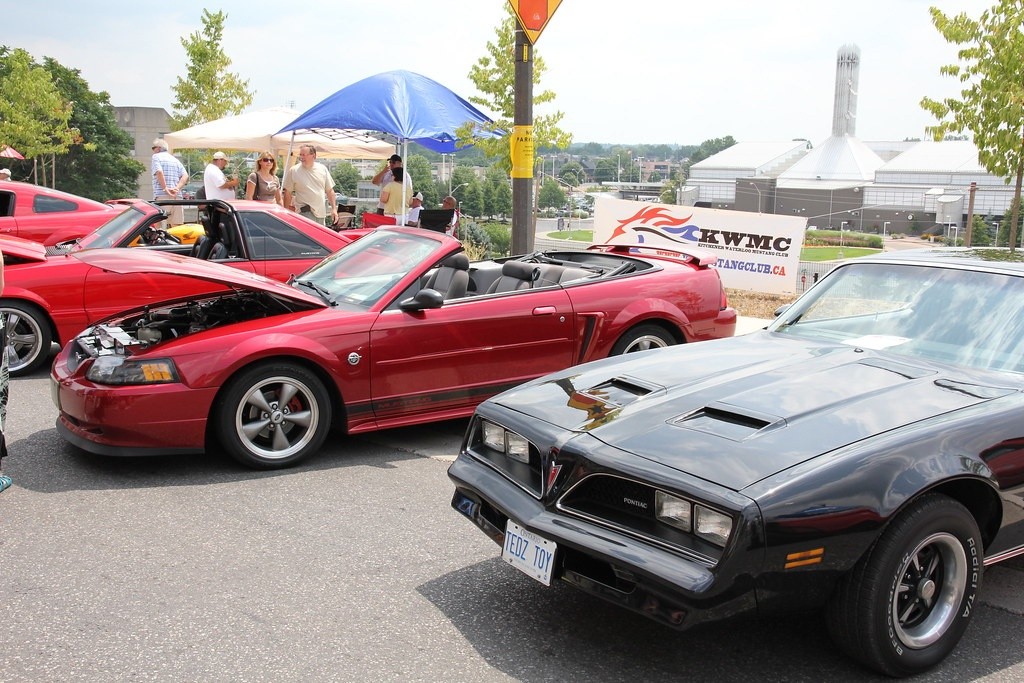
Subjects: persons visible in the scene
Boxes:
[247,150,283,206]
[204,151,238,201]
[380,167,413,225]
[151,138,188,229]
[443,196,459,237]
[404,190,424,227]
[0,168,11,181]
[283,145,338,226]
[372,154,412,216]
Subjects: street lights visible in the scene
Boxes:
[451,182,469,197]
[883,222,891,254]
[839,221,849,260]
[749,181,762,214]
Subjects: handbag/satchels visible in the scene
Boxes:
[243,172,260,200]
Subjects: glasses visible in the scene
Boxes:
[263,158,274,162]
[151,146,161,151]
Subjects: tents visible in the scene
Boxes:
[269,69,510,227]
[163,107,396,194]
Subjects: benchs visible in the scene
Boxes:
[470,263,593,295]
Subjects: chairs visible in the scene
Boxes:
[423,254,470,300]
[327,204,356,232]
[207,222,230,259]
[417,208,460,239]
[486,260,541,295]
[362,212,396,228]
[189,216,213,259]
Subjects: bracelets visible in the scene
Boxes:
[163,186,167,191]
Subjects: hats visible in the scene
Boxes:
[213,152,229,160]
[412,191,423,201]
[0,168,12,177]
[387,154,402,162]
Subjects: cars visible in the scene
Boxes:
[445,250,1024,678]
[0,173,149,242]
[46,223,740,467]
[0,200,425,391]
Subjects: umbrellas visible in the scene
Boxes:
[0,144,24,159]
